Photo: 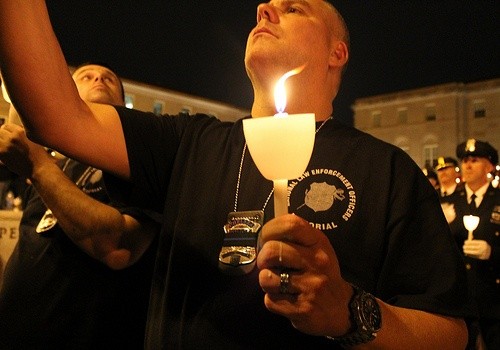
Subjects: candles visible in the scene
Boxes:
[468,215,473,242]
[273,58,307,219]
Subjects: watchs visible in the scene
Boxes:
[325,281,383,347]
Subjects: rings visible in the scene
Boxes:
[279,272,290,296]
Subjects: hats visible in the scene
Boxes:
[456,139,498,165]
[423,167,438,178]
[431,158,459,170]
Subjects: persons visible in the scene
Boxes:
[0,62,162,350]
[421,139,500,350]
[0,0,479,350]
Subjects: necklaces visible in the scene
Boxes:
[219,116,333,264]
[36,158,92,233]
